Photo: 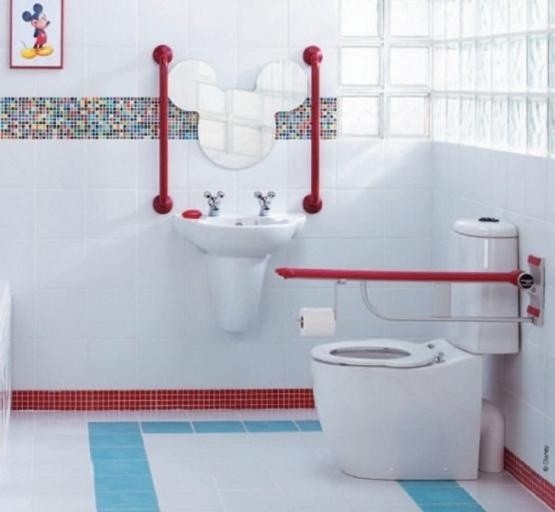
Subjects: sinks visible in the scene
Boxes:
[173,212,307,332]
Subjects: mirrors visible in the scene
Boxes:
[167,59,309,170]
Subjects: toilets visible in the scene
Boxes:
[310,217,520,480]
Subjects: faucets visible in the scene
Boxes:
[204,191,223,216]
[255,190,275,216]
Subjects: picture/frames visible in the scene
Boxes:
[10,0,64,68]
